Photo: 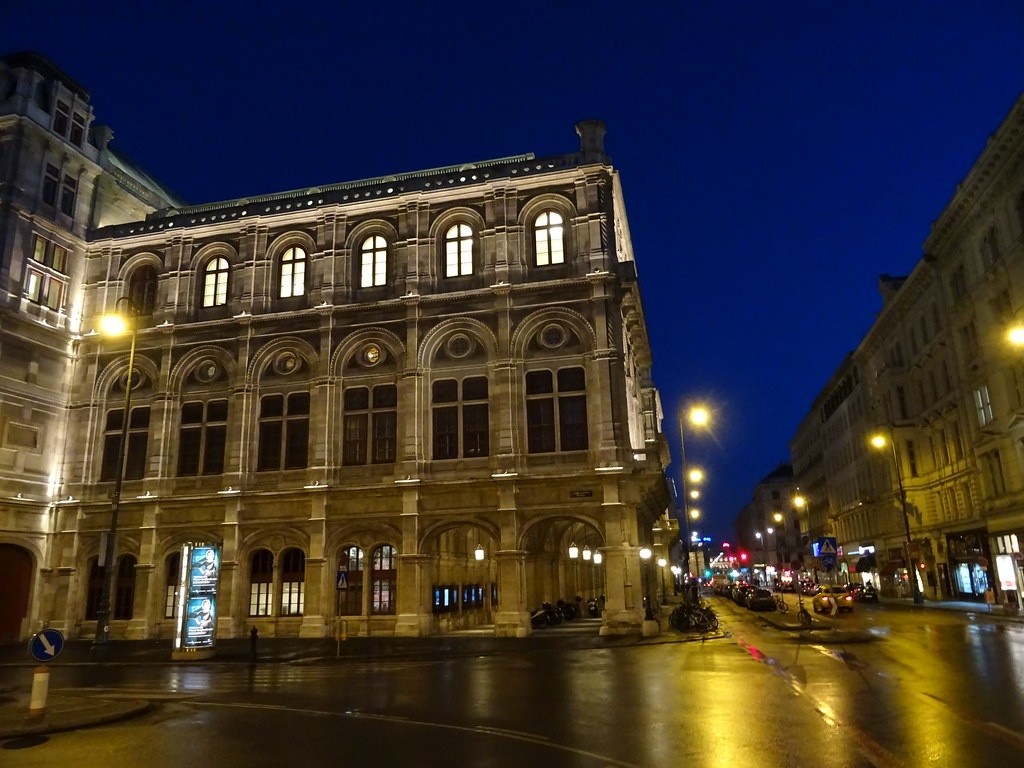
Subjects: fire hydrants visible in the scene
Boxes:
[250,624,258,647]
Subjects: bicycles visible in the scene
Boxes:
[668,602,718,634]
[773,594,788,613]
[795,599,812,625]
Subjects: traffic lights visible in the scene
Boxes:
[704,570,711,579]
[739,551,750,564]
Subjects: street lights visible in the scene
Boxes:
[792,496,818,584]
[88,297,139,661]
[679,401,714,606]
[870,423,924,604]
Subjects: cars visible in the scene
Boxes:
[775,580,878,613]
[703,583,775,611]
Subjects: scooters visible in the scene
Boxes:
[585,599,599,618]
[528,595,582,629]
